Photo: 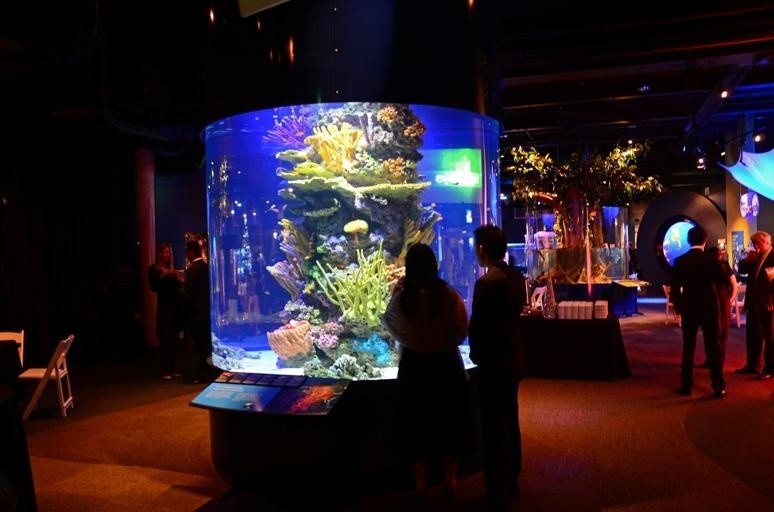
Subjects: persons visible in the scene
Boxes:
[670,226,729,398]
[468,224,529,500]
[629,242,640,275]
[148,243,184,379]
[698,237,734,368]
[383,243,468,491]
[736,232,774,381]
[166,241,220,384]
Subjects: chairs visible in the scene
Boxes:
[725,283,748,330]
[17,332,76,422]
[0,329,26,368]
[662,284,682,329]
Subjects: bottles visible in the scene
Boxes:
[557,300,608,320]
[531,229,558,250]
[524,276,530,305]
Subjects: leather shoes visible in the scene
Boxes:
[715,390,725,398]
[737,368,763,375]
[760,372,771,380]
[675,385,691,396]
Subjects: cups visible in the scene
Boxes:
[177,269,185,277]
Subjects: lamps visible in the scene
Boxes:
[684,115,773,171]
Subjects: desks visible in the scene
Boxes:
[521,310,633,386]
